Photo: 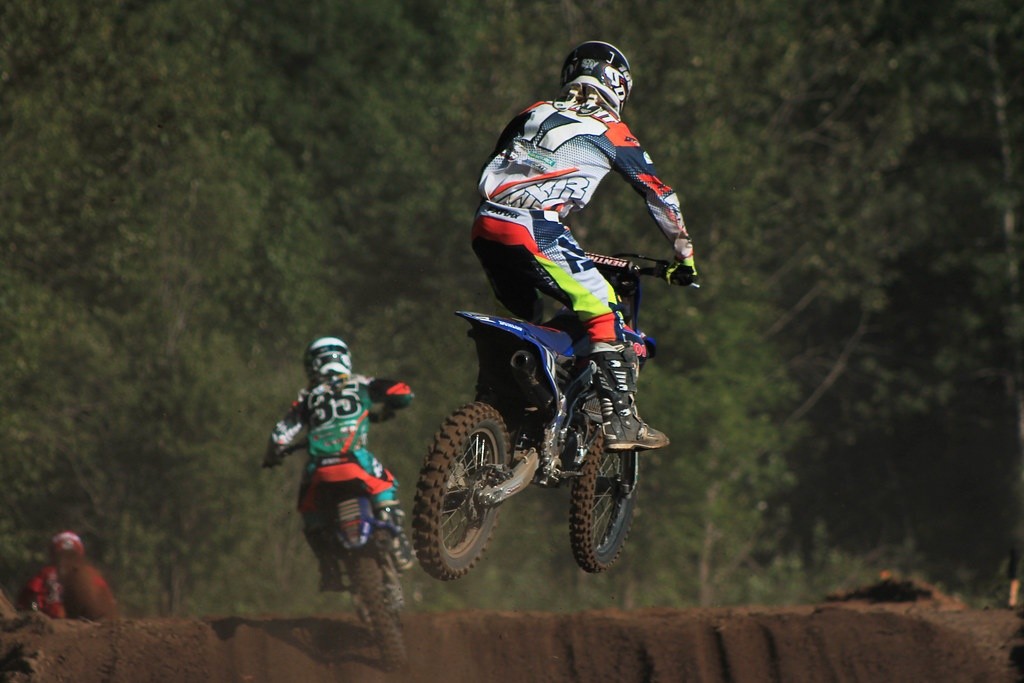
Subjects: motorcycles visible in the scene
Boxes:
[412,251,701,582]
[263,411,409,672]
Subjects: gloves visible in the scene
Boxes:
[665,254,698,287]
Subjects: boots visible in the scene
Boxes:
[587,348,670,453]
[377,504,418,571]
[304,522,342,592]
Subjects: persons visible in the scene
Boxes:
[29,530,115,618]
[263,336,417,593]
[470,40,699,451]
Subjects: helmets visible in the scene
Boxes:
[304,337,352,386]
[49,531,83,556]
[559,41,633,121]
